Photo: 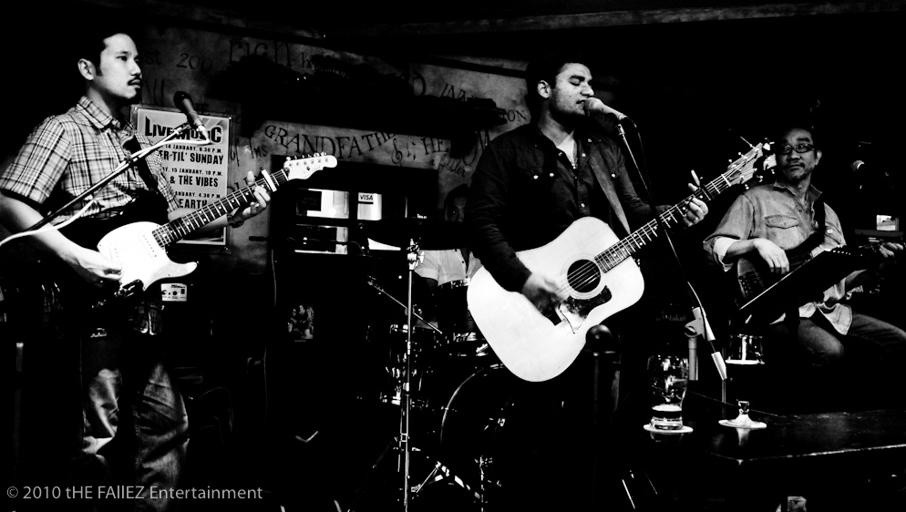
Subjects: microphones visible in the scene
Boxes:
[171,89,215,145]
[582,92,630,130]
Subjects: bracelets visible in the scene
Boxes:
[228,210,242,228]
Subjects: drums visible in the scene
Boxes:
[380,324,438,406]
[410,332,563,512]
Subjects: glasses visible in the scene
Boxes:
[779,144,819,153]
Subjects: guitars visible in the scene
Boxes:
[61,151,338,328]
[465,136,777,383]
[734,236,905,308]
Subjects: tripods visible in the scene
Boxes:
[349,247,484,512]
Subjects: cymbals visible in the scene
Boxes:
[365,216,465,249]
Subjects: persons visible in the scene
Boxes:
[466,45,713,512]
[704,117,906,413]
[1,23,276,511]
